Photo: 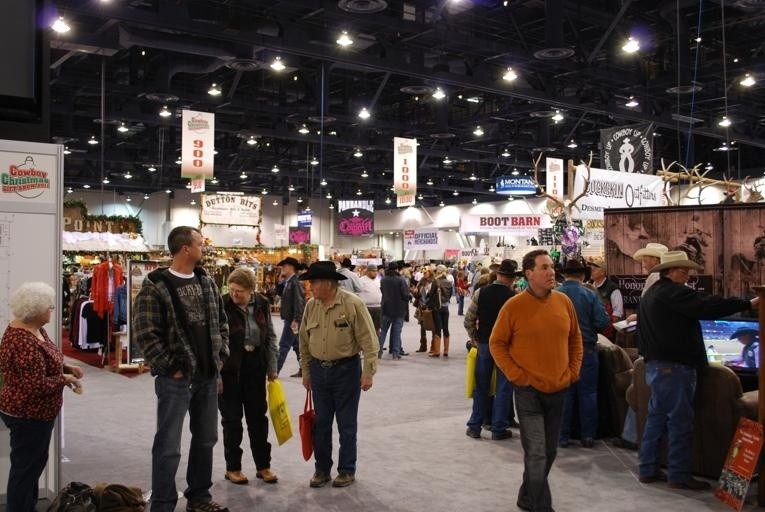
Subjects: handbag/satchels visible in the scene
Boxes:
[298,408,318,462]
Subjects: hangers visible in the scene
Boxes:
[77,292,96,308]
[121,277,128,289]
[97,257,121,271]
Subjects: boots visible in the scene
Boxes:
[442,334,450,356]
[428,334,441,357]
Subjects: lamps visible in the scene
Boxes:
[50,0,71,35]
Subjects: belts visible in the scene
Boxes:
[311,353,360,369]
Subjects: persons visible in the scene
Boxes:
[633,251,761,492]
[296,262,379,489]
[0,281,83,512]
[489,250,584,512]
[217,267,280,485]
[273,242,761,441]
[130,226,231,512]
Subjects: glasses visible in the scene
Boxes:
[48,304,54,311]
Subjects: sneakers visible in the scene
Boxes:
[332,470,356,488]
[308,470,332,488]
[185,495,231,512]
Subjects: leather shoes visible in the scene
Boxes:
[612,437,638,451]
[465,426,482,439]
[666,474,713,490]
[638,470,668,484]
[255,466,278,483]
[224,469,248,485]
[491,430,513,440]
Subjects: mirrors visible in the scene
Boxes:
[129,260,161,363]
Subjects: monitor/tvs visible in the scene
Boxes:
[698,319,760,369]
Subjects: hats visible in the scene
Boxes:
[367,264,377,271]
[559,259,592,274]
[488,258,524,278]
[728,325,760,342]
[397,259,410,270]
[296,260,349,282]
[388,261,398,270]
[340,258,355,271]
[632,242,669,262]
[590,260,607,271]
[648,250,701,274]
[276,257,304,270]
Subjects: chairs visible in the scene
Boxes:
[693,362,759,481]
[627,357,668,462]
[596,333,642,436]
